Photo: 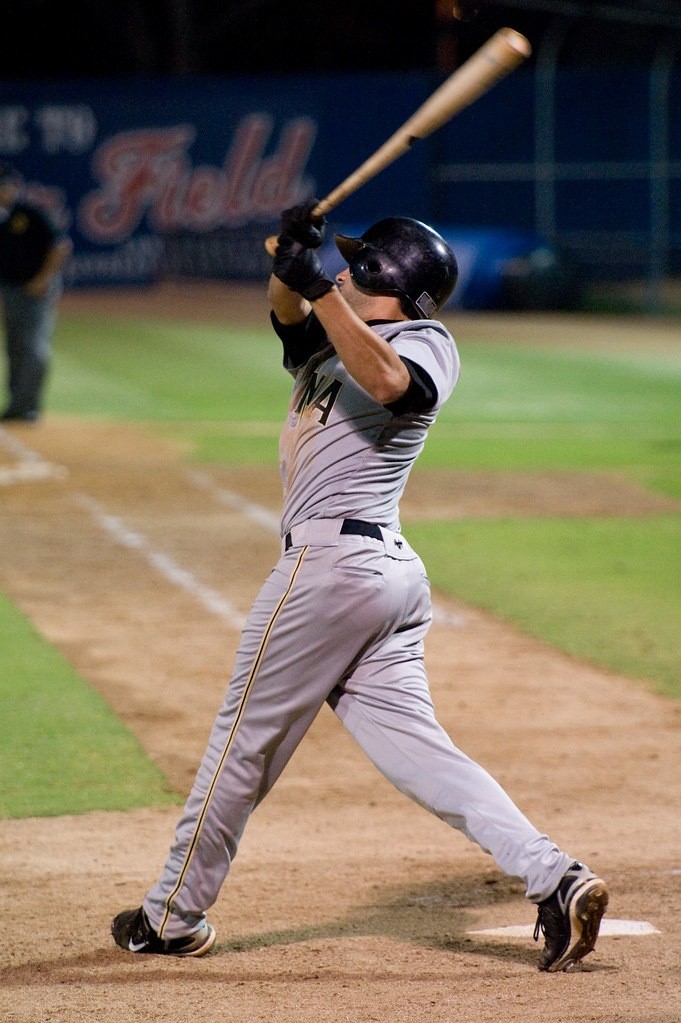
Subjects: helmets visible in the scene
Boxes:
[333,216,458,320]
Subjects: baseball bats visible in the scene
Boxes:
[264,27,533,259]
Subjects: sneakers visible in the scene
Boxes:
[532,861,609,973]
[110,905,216,958]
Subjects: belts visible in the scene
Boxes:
[285,519,383,551]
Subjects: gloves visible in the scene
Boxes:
[281,197,328,256]
[273,231,335,302]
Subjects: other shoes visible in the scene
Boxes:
[0,410,40,424]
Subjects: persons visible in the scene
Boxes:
[111,200,610,972]
[0,171,72,423]
